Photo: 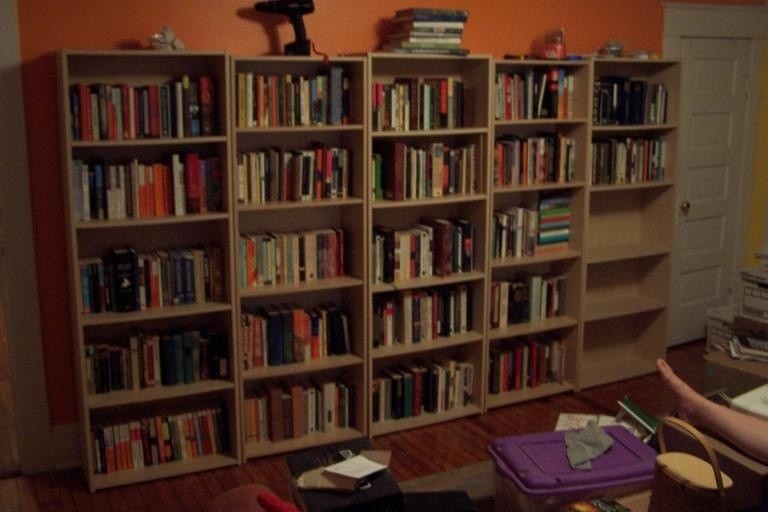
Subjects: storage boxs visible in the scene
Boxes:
[697,315,768,397]
[486,424,659,512]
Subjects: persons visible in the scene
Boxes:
[655,357,768,465]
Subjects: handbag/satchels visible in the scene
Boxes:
[649,416,734,512]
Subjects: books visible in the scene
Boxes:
[590,136,666,183]
[83,327,230,394]
[556,396,665,450]
[92,402,232,475]
[494,133,575,187]
[242,375,348,441]
[371,215,474,346]
[240,303,350,370]
[495,67,571,120]
[373,358,473,422]
[235,66,352,202]
[594,71,666,125]
[72,153,222,220]
[378,7,470,57]
[80,245,225,314]
[722,335,768,361]
[491,270,566,394]
[493,193,574,259]
[70,74,220,141]
[372,78,483,201]
[238,228,343,288]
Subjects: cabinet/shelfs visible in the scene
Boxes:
[487,58,591,410]
[580,56,682,390]
[369,55,490,436]
[54,48,243,493]
[231,57,368,457]
